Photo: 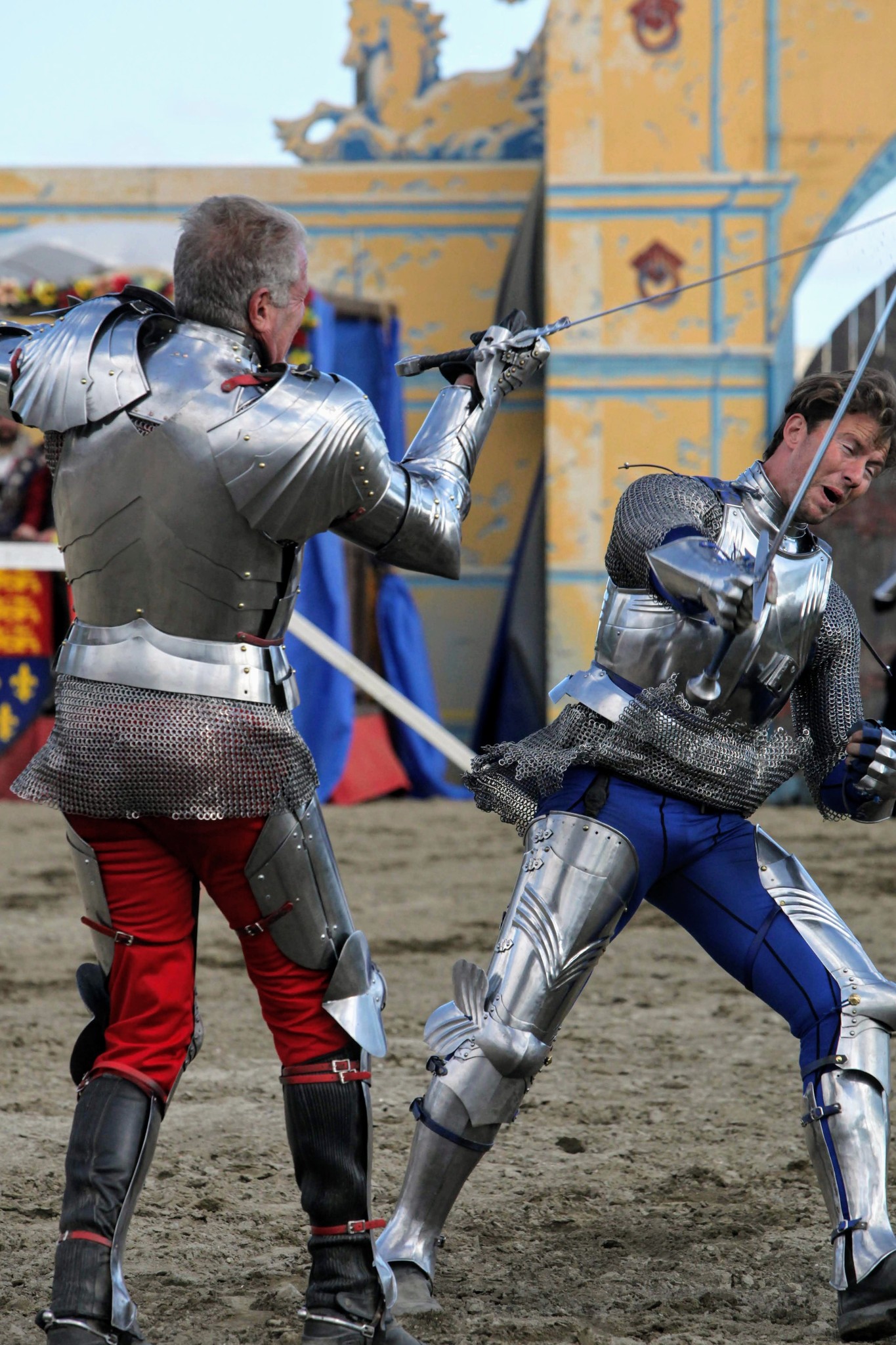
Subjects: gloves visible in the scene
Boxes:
[439,307,535,387]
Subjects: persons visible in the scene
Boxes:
[374,366,896,1341]
[0,197,554,1345]
[0,370,64,548]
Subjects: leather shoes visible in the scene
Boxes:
[836,1249,896,1343]
[384,1261,442,1319]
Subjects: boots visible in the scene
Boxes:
[282,1044,423,1345]
[36,1075,162,1345]
